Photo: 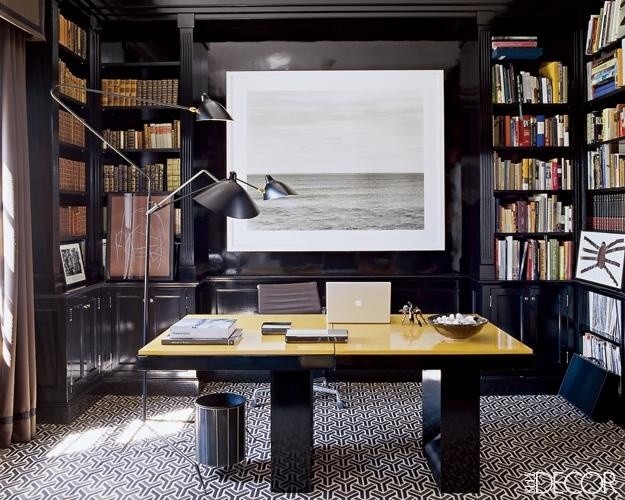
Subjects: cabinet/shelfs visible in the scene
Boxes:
[579,5,622,413]
[480,16,579,393]
[47,5,99,422]
[100,3,193,397]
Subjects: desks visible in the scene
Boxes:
[121,315,534,494]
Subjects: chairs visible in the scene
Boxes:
[246,282,347,407]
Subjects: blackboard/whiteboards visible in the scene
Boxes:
[107,193,174,283]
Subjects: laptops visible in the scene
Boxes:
[325,282,391,323]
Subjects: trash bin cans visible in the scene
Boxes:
[195,391,247,466]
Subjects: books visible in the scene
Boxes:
[160,317,245,346]
[58,13,182,270]
[491,0,625,376]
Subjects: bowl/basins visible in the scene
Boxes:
[426,311,488,340]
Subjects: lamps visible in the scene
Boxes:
[49,91,299,422]
